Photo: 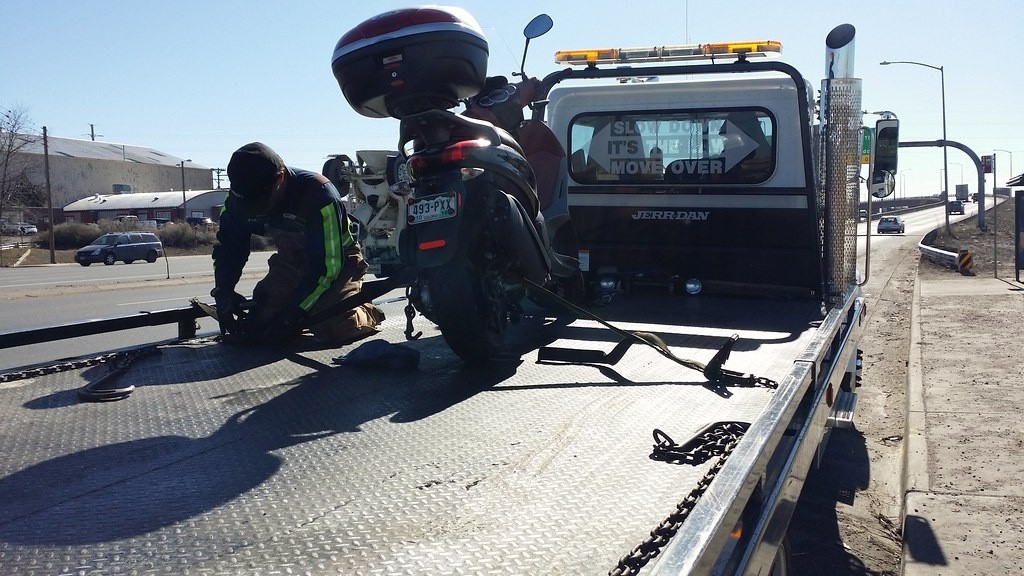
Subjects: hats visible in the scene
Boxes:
[226,142,280,222]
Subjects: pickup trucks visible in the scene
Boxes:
[110,215,158,230]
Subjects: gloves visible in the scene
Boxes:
[210,285,249,335]
[261,306,307,346]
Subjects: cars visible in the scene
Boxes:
[948,200,966,215]
[5,221,38,236]
[857,210,867,217]
[876,215,906,235]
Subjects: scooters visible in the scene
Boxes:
[332,4,587,367]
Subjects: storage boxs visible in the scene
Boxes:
[331,5,489,118]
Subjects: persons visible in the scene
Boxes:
[211,142,386,348]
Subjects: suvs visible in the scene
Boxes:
[186,216,217,228]
[74,231,163,267]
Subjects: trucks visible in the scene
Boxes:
[955,184,971,201]
[972,192,979,203]
[0,24,903,576]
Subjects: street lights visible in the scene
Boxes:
[898,168,912,199]
[993,149,1012,179]
[939,169,945,192]
[878,60,949,233]
[950,163,963,184]
[175,159,193,222]
